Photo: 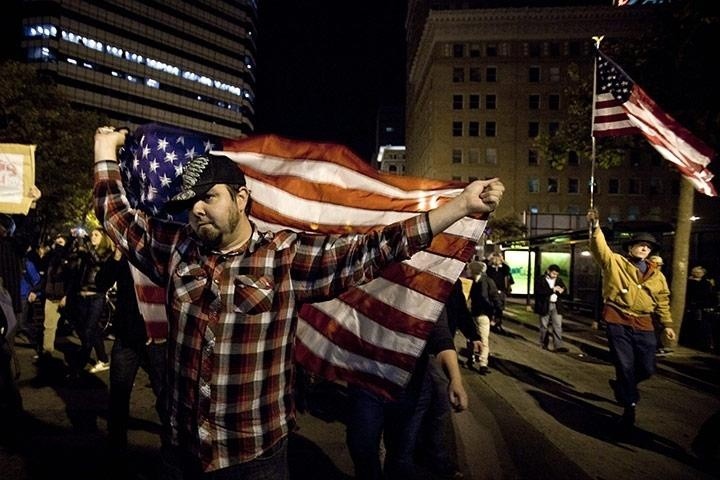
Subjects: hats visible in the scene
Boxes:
[171,152,246,209]
[622,232,663,252]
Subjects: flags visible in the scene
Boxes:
[592,46,718,197]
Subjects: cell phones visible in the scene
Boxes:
[76,227,86,236]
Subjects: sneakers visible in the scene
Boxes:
[480,365,492,374]
[32,348,52,359]
[89,360,110,373]
[556,347,569,352]
[622,402,636,425]
[608,378,621,403]
